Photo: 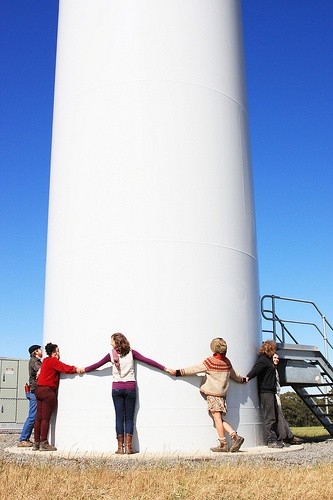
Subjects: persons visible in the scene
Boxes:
[33,343,79,451]
[80,333,174,453]
[18,343,44,447]
[246,341,302,448]
[177,338,248,453]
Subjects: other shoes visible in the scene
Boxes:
[230,436,244,452]
[39,440,57,451]
[33,441,39,451]
[211,442,229,452]
[279,440,290,447]
[268,441,284,448]
[17,440,33,447]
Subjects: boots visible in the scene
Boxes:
[125,433,136,454]
[115,434,124,454]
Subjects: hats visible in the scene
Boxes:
[28,345,41,354]
